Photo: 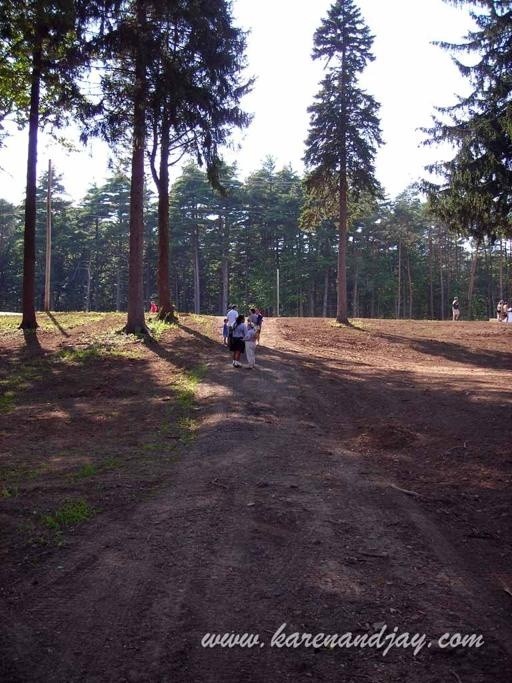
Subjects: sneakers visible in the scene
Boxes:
[233,362,255,369]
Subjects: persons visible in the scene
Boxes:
[506,307,512,322]
[451,296,461,320]
[222,305,264,370]
[150,300,157,313]
[497,299,504,322]
[503,301,509,322]
